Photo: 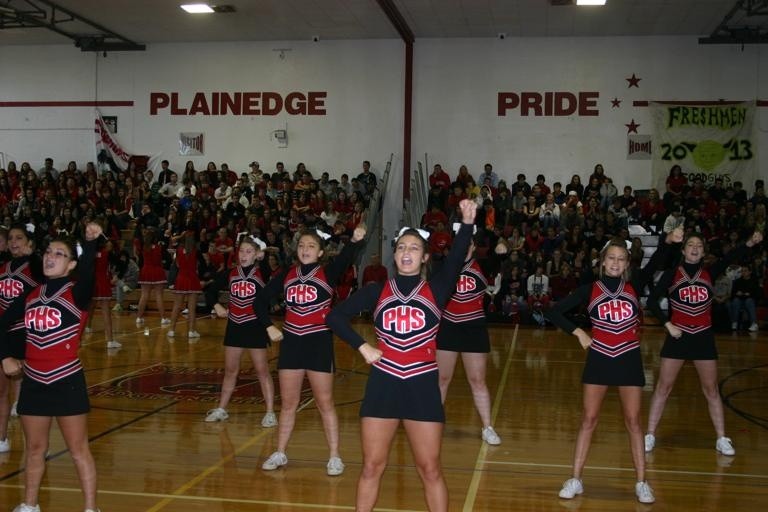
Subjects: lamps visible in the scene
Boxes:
[180,5,216,15]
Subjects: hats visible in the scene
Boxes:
[569,191,577,196]
[249,161,259,167]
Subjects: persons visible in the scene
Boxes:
[335,222,353,238]
[323,160,378,199]
[525,229,545,265]
[546,227,557,248]
[0,157,104,222]
[466,181,480,198]
[430,164,450,188]
[447,187,468,208]
[553,182,565,203]
[456,164,476,185]
[267,255,282,279]
[168,262,176,289]
[428,222,453,261]
[133,232,171,325]
[494,192,511,225]
[481,261,504,314]
[325,199,478,512]
[562,191,583,214]
[450,205,463,224]
[735,233,767,339]
[478,164,499,187]
[282,232,299,256]
[429,186,446,208]
[527,266,550,310]
[533,174,551,196]
[0,222,103,512]
[427,223,502,445]
[497,179,510,192]
[644,232,735,456]
[539,193,560,228]
[547,250,569,279]
[114,251,141,313]
[265,233,282,253]
[0,226,8,265]
[104,161,198,232]
[330,230,345,242]
[513,189,527,219]
[203,230,278,427]
[198,252,220,310]
[481,185,493,201]
[631,237,643,270]
[565,175,584,201]
[109,240,129,277]
[364,255,388,285]
[322,202,339,225]
[326,249,346,278]
[585,176,600,198]
[249,160,323,226]
[200,162,247,228]
[165,237,202,337]
[586,197,600,225]
[563,223,587,253]
[253,227,365,475]
[591,164,607,180]
[478,199,503,235]
[562,204,584,228]
[570,249,586,275]
[327,239,344,255]
[216,227,234,252]
[546,227,684,504]
[423,205,449,226]
[521,195,540,234]
[85,239,122,348]
[349,200,366,227]
[599,164,767,232]
[489,224,510,271]
[0,223,42,452]
[206,241,224,272]
[508,226,526,262]
[588,225,610,261]
[512,174,531,196]
[503,265,526,321]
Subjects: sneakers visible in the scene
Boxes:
[10,401,20,417]
[211,309,216,313]
[716,437,735,455]
[166,330,175,337]
[161,317,171,324]
[749,322,758,331]
[123,285,132,294]
[136,316,144,322]
[188,330,200,337]
[262,451,288,469]
[183,308,188,313]
[112,303,123,312]
[107,340,121,348]
[12,502,41,512]
[644,433,655,451]
[86,327,96,332]
[1,437,9,452]
[635,482,655,503]
[481,426,502,445]
[558,477,584,499]
[327,457,344,475]
[205,408,228,422]
[261,412,278,427]
[85,506,102,512]
